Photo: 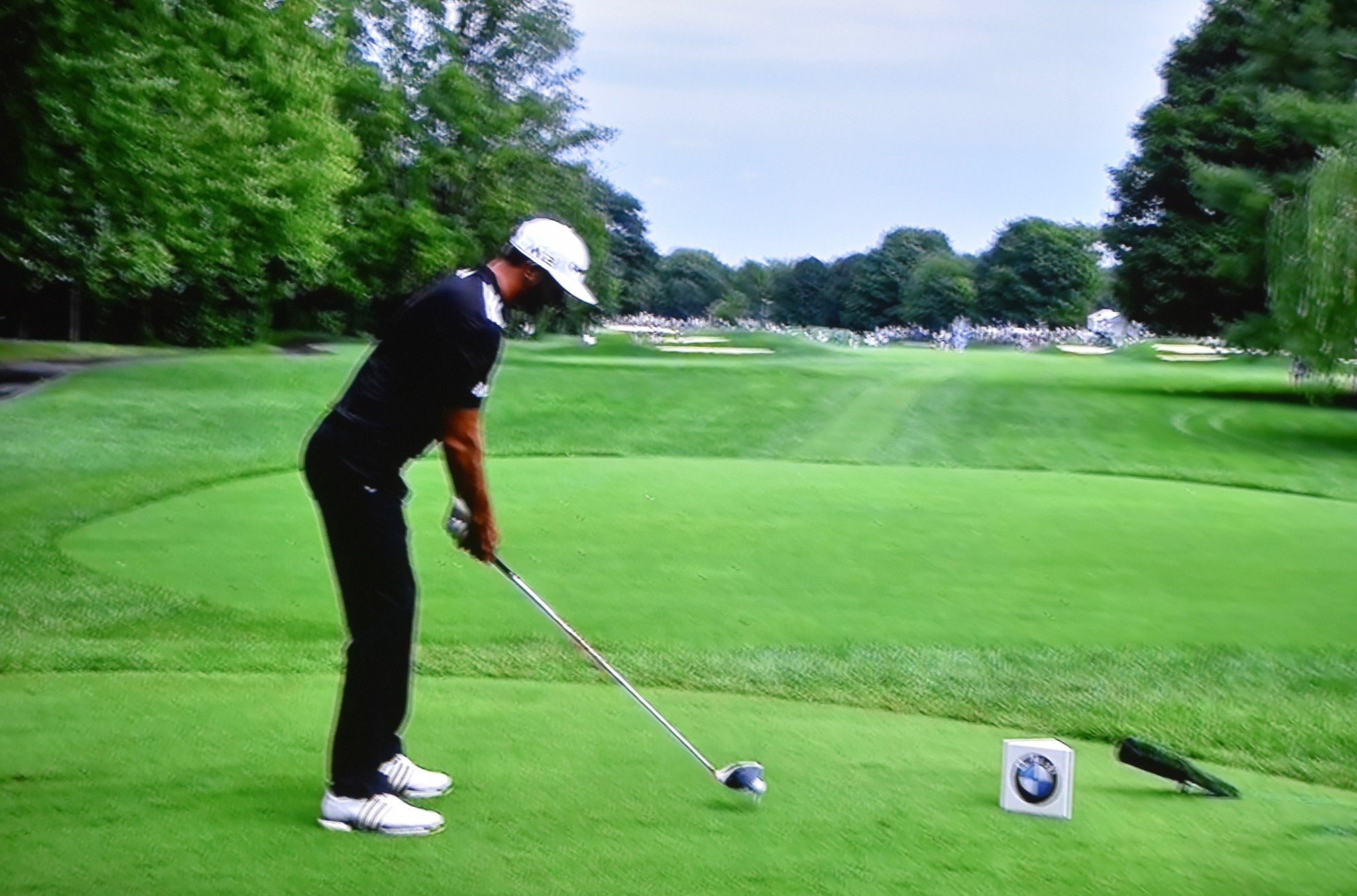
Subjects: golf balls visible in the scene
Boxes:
[746,776,764,793]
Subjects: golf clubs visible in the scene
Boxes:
[442,493,764,790]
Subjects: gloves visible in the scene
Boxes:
[446,496,472,541]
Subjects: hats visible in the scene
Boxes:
[509,217,599,305]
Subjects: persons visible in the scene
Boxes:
[301,214,604,837]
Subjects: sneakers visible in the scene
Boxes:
[378,753,456,798]
[316,789,447,836]
